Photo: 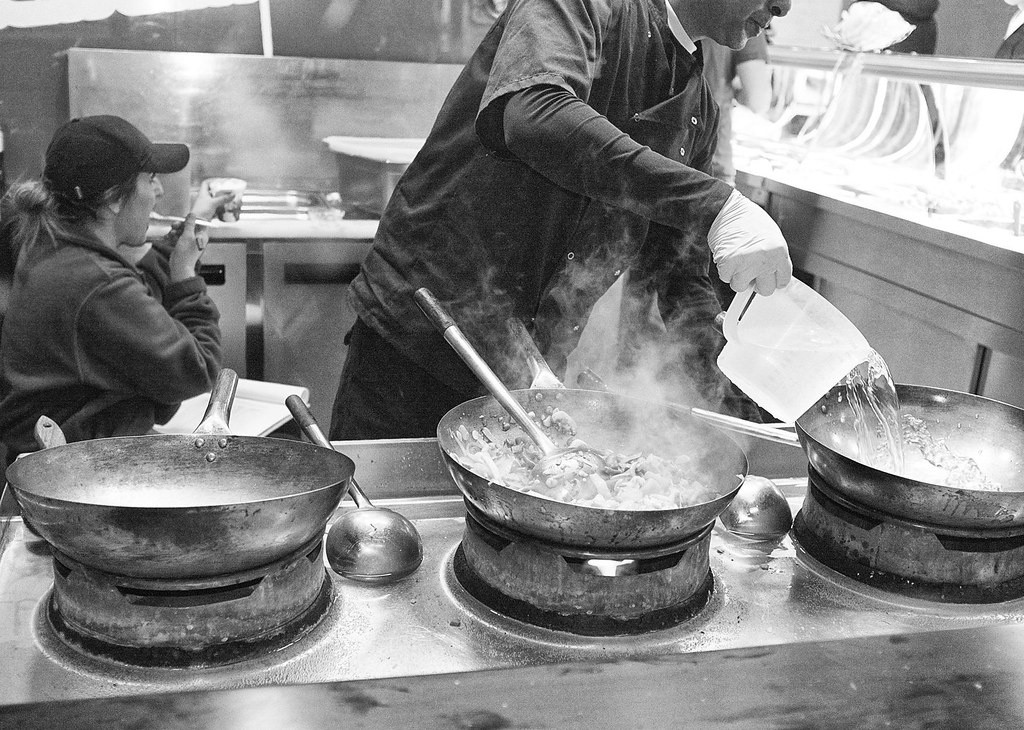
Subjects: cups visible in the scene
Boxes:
[210,177,246,223]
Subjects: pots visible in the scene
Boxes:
[664,382,1024,527]
[436,316,750,551]
[5,368,357,577]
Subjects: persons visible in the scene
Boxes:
[328,1,794,442]
[0,115,236,466]
[994,0,1024,60]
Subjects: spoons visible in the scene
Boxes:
[148,211,235,230]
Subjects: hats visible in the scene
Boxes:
[42,116,190,199]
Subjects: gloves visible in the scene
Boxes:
[708,187,792,297]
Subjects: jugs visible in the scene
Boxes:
[717,271,874,423]
